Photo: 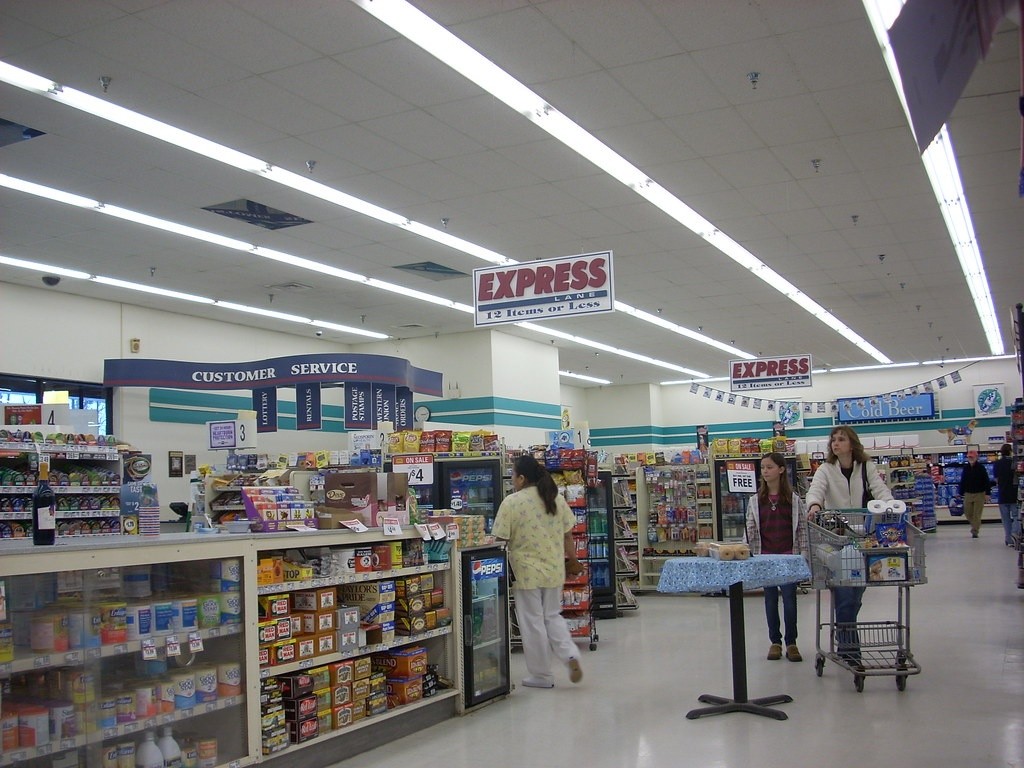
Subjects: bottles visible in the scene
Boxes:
[449,486,495,504]
[157,727,182,768]
[697,471,713,538]
[470,559,504,598]
[474,652,499,695]
[414,487,431,506]
[137,733,163,768]
[723,517,745,538]
[588,491,606,508]
[459,503,495,532]
[588,537,609,560]
[591,563,610,587]
[722,494,747,514]
[589,511,607,534]
[472,608,498,647]
[8,412,23,425]
[32,463,55,545]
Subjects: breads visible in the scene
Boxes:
[694,544,709,557]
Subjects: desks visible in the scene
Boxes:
[656,553,813,721]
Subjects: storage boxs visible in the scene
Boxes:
[316,471,409,530]
[254,553,453,758]
[243,488,315,522]
[947,496,964,516]
[863,553,910,581]
[427,509,494,549]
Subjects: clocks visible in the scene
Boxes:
[414,406,431,422]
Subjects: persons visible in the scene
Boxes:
[490,454,584,689]
[956,450,991,538]
[993,443,1021,547]
[742,425,895,673]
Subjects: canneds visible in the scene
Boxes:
[0,558,243,754]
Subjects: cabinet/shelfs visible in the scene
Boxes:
[0,449,125,537]
[203,468,289,536]
[241,486,318,533]
[863,441,1015,525]
[610,465,725,598]
[496,445,599,651]
[875,463,937,533]
[0,527,462,768]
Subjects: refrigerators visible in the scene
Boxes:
[585,471,618,619]
[713,457,798,543]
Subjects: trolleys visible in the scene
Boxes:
[802,508,928,693]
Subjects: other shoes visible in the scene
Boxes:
[972,534,978,538]
[837,648,863,666]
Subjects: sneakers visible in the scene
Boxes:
[786,645,803,661]
[567,656,583,684]
[767,644,783,660]
[522,676,556,688]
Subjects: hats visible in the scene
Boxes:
[967,450,977,457]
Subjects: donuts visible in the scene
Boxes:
[708,543,750,560]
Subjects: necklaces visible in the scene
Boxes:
[767,495,779,511]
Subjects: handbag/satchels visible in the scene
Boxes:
[862,489,875,508]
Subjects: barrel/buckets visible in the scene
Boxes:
[97,741,137,768]
[11,559,241,652]
[65,644,244,734]
[173,730,217,768]
[0,700,76,752]
